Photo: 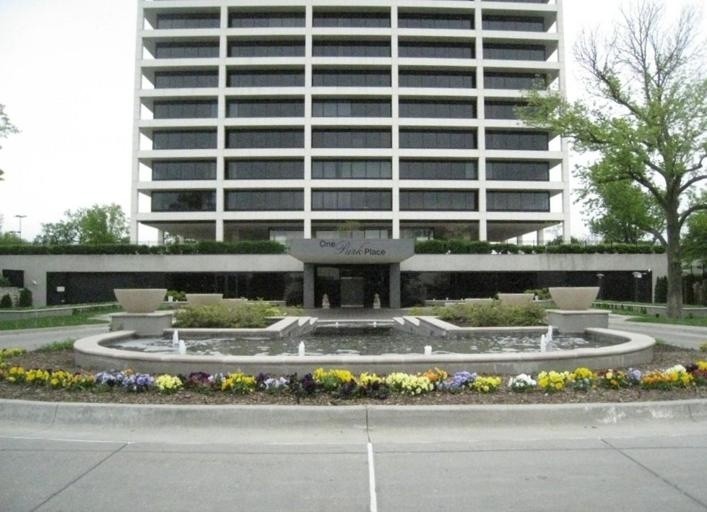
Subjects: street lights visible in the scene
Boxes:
[597,272,604,298]
[15,215,27,239]
[632,271,642,301]
